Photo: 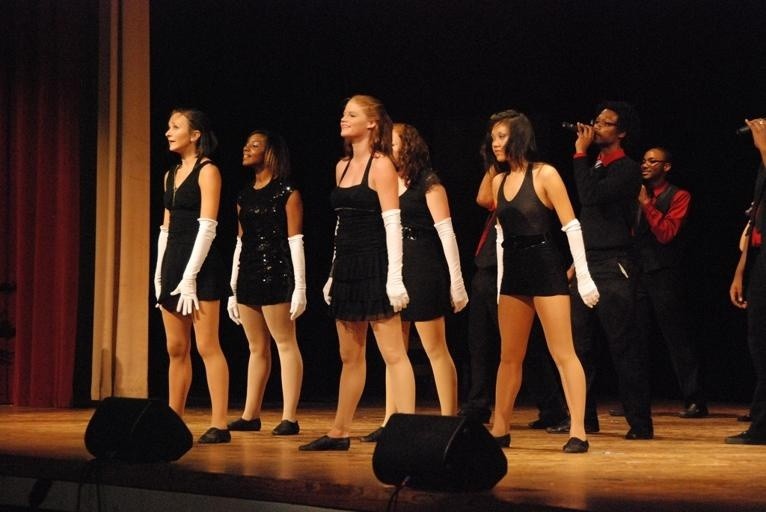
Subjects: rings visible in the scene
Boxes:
[759,121,763,125]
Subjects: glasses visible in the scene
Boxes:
[590,119,616,127]
[640,159,667,163]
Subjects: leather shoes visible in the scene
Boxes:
[458,407,492,425]
[626,423,655,440]
[360,424,384,444]
[610,406,626,416]
[724,429,766,444]
[229,417,261,432]
[298,433,352,452]
[564,435,589,454]
[492,430,513,449]
[678,402,709,419]
[199,426,232,444]
[272,420,300,437]
[528,413,601,434]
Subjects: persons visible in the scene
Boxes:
[361,122,470,442]
[545,100,654,439]
[153,108,234,443]
[725,118,766,445]
[737,201,757,422]
[299,94,416,451]
[609,146,709,418]
[457,145,570,429]
[489,110,600,453]
[226,129,308,435]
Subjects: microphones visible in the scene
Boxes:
[735,118,766,137]
[562,121,596,136]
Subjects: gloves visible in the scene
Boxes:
[562,218,601,307]
[432,217,468,313]
[227,235,244,326]
[287,234,308,320]
[381,208,410,311]
[169,218,219,315]
[153,224,170,311]
